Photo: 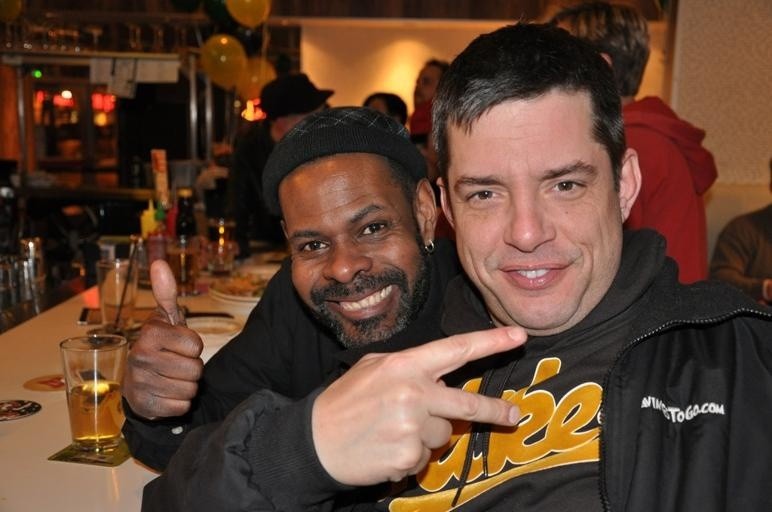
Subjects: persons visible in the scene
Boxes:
[142,13,770,512]
[708,159,772,308]
[222,73,334,251]
[549,1,719,286]
[410,58,454,154]
[364,92,407,124]
[122,106,483,475]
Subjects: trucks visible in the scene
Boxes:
[201,34,246,91]
[238,57,276,101]
[227,1,270,27]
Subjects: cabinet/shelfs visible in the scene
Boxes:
[174,188,198,294]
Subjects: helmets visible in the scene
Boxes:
[207,264,280,300]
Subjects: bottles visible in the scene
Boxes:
[255,69,335,115]
[261,106,428,214]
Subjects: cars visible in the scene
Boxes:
[59,333,130,451]
[205,214,236,276]
[95,258,139,334]
